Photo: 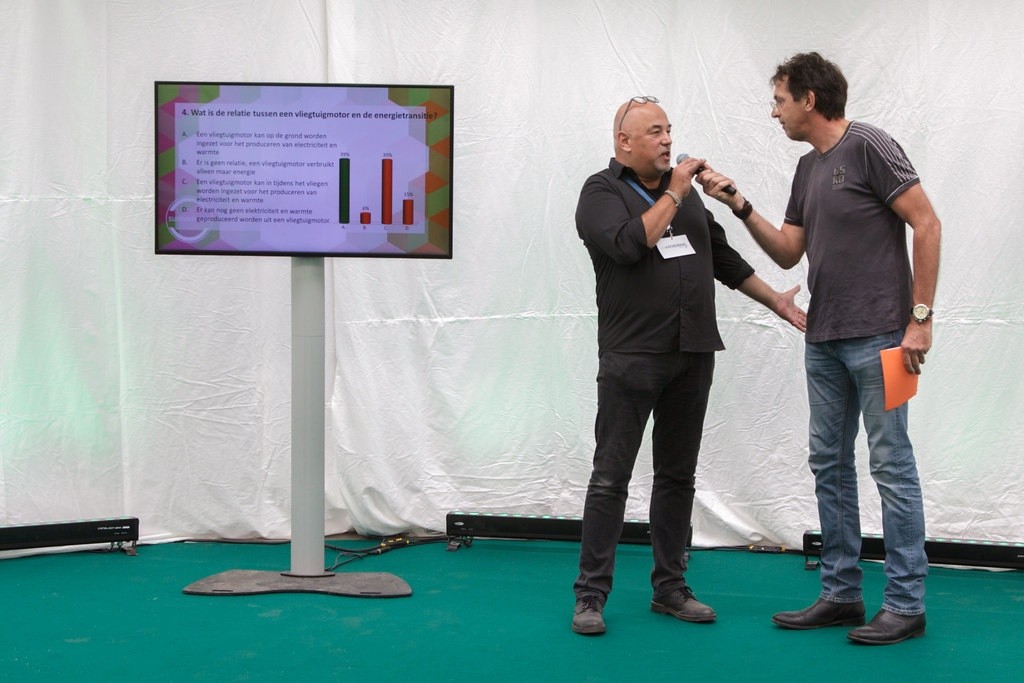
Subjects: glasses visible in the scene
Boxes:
[769,92,799,112]
[619,96,659,131]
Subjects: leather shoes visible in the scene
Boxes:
[771,598,865,629]
[847,609,926,644]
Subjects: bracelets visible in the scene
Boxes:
[664,188,684,209]
[731,196,753,219]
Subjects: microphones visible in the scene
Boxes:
[676,153,737,196]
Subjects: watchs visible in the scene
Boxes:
[909,303,935,324]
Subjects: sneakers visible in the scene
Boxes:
[572,596,606,633]
[651,586,716,621]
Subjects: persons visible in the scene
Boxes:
[694,51,941,645]
[572,95,807,634]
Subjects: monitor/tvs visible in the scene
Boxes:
[155,79,454,258]
